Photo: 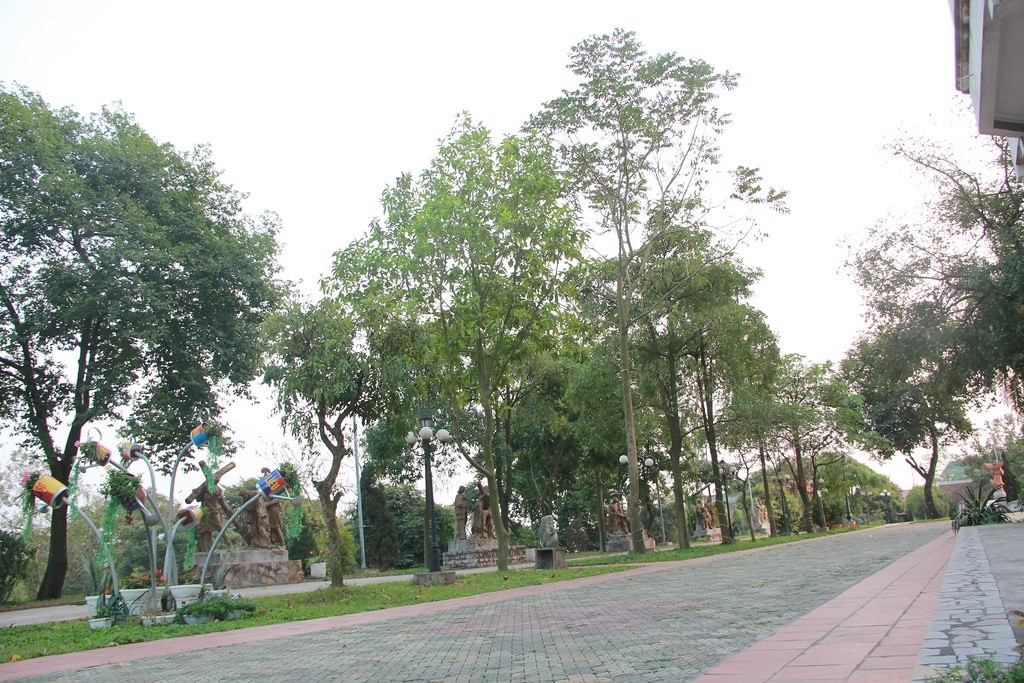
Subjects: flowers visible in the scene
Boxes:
[72,437,97,467]
[19,469,41,491]
[95,587,109,617]
[304,549,323,564]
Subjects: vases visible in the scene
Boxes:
[309,562,326,578]
[32,472,69,513]
[88,617,111,630]
[89,442,111,466]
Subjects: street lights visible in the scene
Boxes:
[619,455,654,499]
[405,427,450,573]
[418,410,433,570]
[771,474,790,536]
[880,489,891,524]
[809,478,825,528]
[718,466,737,540]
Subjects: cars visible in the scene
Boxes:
[588,544,600,552]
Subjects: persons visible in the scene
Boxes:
[989,460,1004,490]
[697,498,715,529]
[609,493,630,532]
[197,474,286,550]
[455,486,493,538]
[753,497,766,522]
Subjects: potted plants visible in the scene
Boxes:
[191,420,235,492]
[134,592,255,627]
[97,466,159,563]
[254,460,303,506]
